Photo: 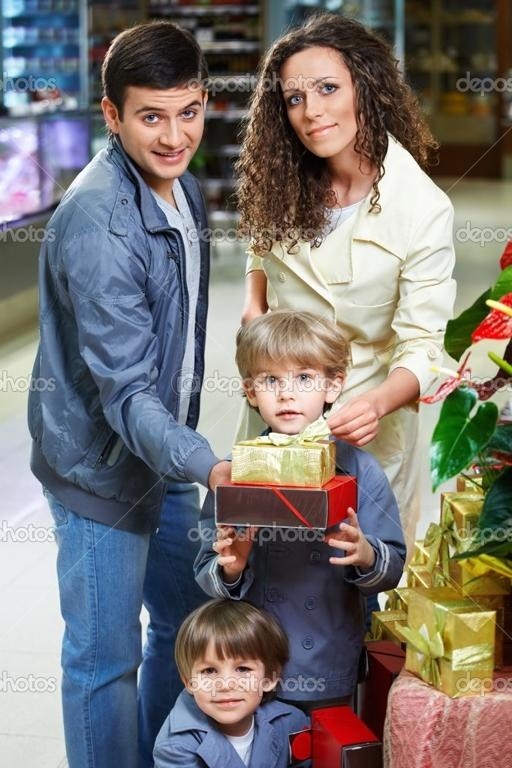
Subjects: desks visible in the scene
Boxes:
[382,665,512,768]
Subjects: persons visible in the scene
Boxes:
[20,20,232,768]
[189,306,407,716]
[152,594,317,767]
[231,13,461,612]
